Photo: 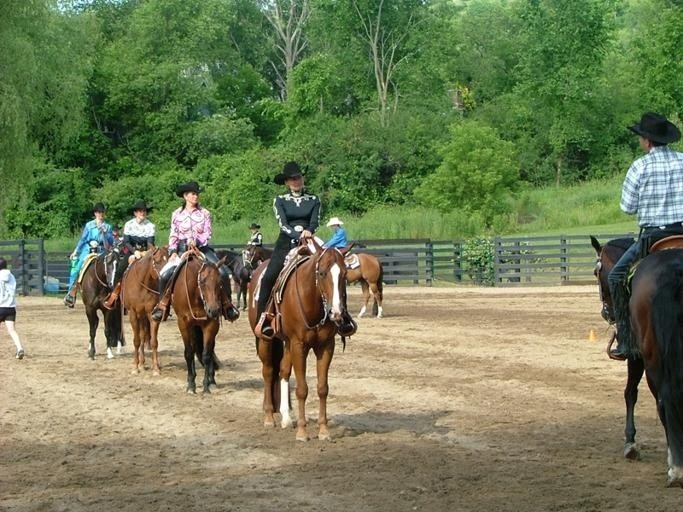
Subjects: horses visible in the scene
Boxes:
[169,247,227,395]
[246,236,356,442]
[214,249,262,312]
[307,233,383,319]
[119,242,170,377]
[78,248,126,361]
[588,233,683,487]
[243,243,274,270]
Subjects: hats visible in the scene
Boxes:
[626,113,681,144]
[248,223,261,229]
[94,202,108,211]
[177,182,201,196]
[326,217,343,227]
[127,201,153,216]
[274,162,308,185]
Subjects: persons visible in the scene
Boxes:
[105,202,156,310]
[253,161,320,338]
[63,203,112,308]
[607,112,682,360]
[111,224,122,242]
[248,223,263,265]
[0,257,24,359]
[324,217,348,249]
[152,183,239,321]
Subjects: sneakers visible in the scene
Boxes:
[151,308,163,320]
[254,322,274,336]
[227,306,239,319]
[340,324,353,333]
[609,343,630,360]
[16,348,24,359]
[104,300,113,308]
[66,294,73,303]
